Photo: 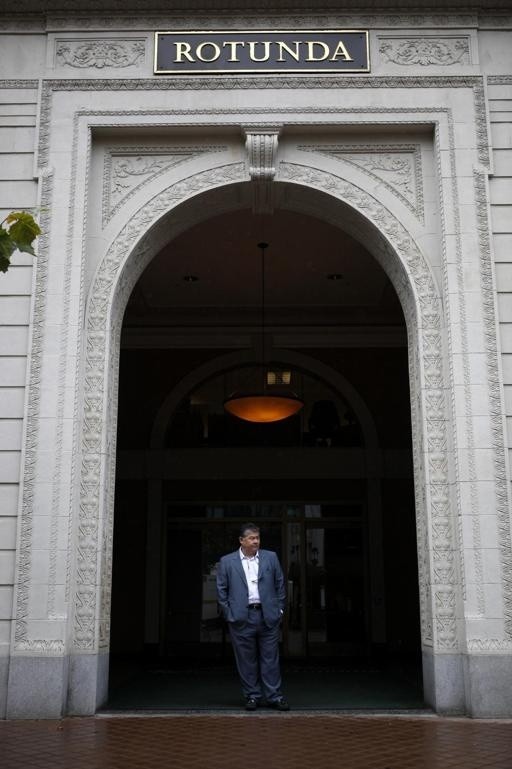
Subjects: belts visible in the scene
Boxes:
[249,604,261,609]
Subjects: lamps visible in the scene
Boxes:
[223,243,304,423]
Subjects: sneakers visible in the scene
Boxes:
[273,700,289,710]
[245,699,257,710]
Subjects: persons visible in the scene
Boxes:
[216,522,292,710]
